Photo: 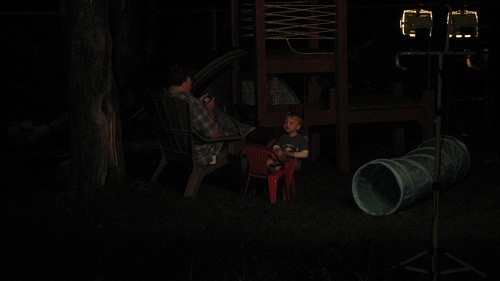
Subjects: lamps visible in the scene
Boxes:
[385,2,490,281]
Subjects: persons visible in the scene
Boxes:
[164,66,272,167]
[263,111,309,201]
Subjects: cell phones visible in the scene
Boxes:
[203,97,210,103]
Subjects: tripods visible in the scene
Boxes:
[386,50,488,281]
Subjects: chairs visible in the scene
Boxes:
[144,92,247,200]
[266,136,299,201]
[239,144,291,205]
